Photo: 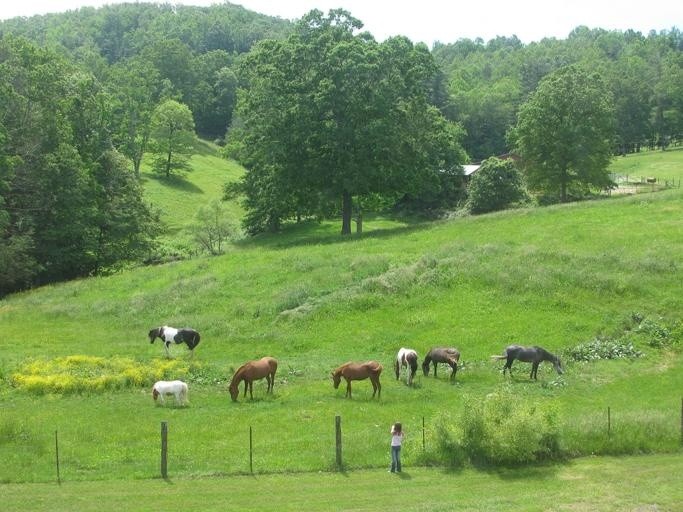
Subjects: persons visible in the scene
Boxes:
[389,422,403,471]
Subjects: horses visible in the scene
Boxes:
[151,378,190,411]
[392,346,418,386]
[228,355,279,404]
[329,360,384,403]
[501,345,565,383]
[422,346,464,381]
[146,324,202,363]
